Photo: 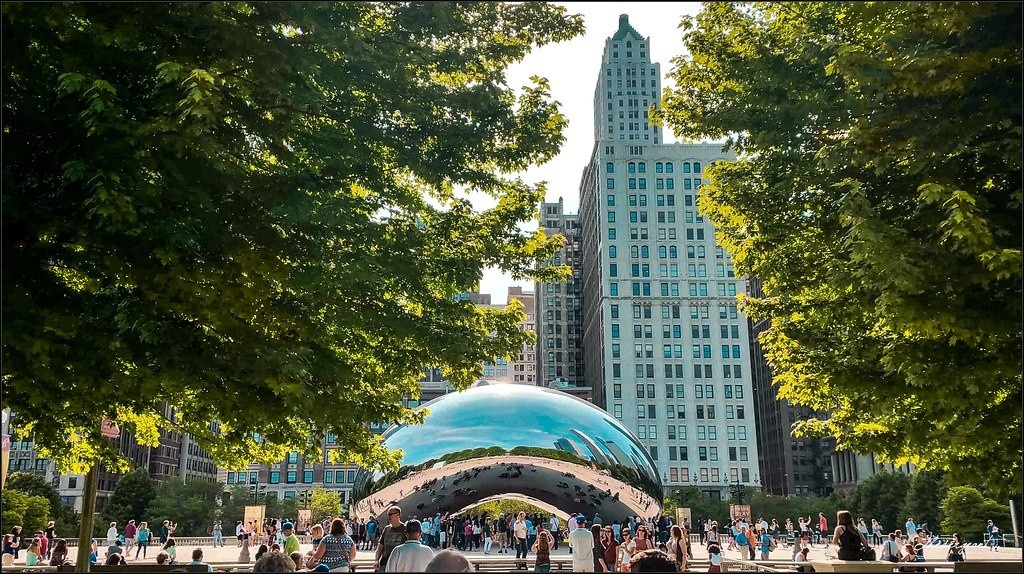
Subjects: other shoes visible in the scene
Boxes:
[990,548,992,551]
[361,547,364,550]
[369,547,373,550]
[505,549,507,553]
[365,547,368,550]
[498,550,502,553]
[995,548,999,552]
[357,546,359,550]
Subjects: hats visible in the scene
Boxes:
[577,516,587,523]
[279,522,294,533]
[316,564,330,572]
[987,520,993,523]
[436,513,441,516]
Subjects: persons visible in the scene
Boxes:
[357,462,660,525]
[1,506,1000,574]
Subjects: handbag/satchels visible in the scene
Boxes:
[889,554,900,564]
[557,527,561,534]
[860,546,876,561]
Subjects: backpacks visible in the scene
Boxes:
[764,535,776,552]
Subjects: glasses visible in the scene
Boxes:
[165,558,171,562]
[622,533,630,536]
[386,512,398,516]
[605,531,612,533]
[638,529,645,532]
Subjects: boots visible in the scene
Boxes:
[569,547,573,553]
